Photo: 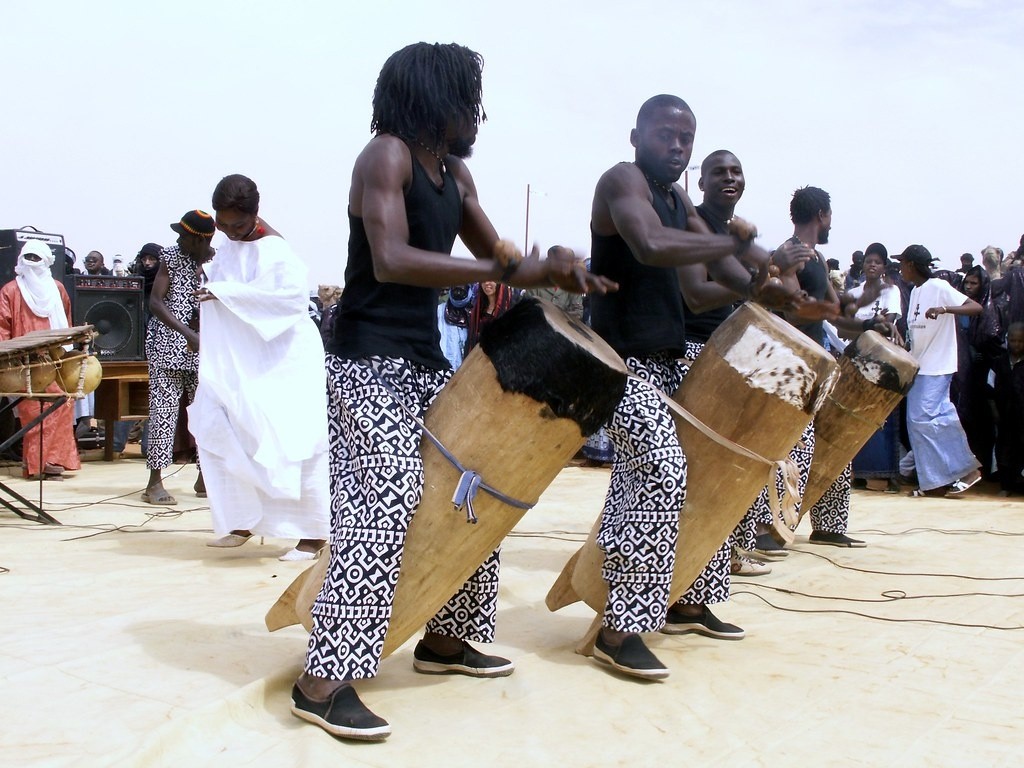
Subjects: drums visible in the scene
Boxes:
[546,298,843,656]
[264,295,630,663]
[763,329,921,548]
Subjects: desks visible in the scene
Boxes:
[94,361,149,463]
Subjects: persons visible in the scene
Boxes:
[826,235,1024,496]
[194,174,332,562]
[434,244,615,470]
[590,93,807,679]
[144,211,229,506]
[890,245,983,498]
[305,280,346,343]
[840,242,903,494]
[292,40,622,743]
[63,243,198,462]
[753,186,868,556]
[685,150,781,578]
[0,239,82,481]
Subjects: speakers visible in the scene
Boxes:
[64,274,146,361]
[0,229,65,287]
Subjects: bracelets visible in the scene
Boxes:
[501,261,512,286]
[941,306,947,313]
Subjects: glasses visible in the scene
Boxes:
[84,257,103,264]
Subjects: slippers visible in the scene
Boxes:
[139,489,178,506]
[908,489,942,497]
[948,476,982,493]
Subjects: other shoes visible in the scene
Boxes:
[756,532,789,557]
[810,530,867,550]
[729,547,772,576]
[592,629,672,678]
[883,482,901,493]
[854,479,867,488]
[42,463,66,482]
[662,606,745,640]
[412,640,515,678]
[288,684,393,742]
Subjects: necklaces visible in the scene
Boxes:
[246,217,263,242]
[792,234,820,261]
[701,205,734,226]
[398,129,447,174]
[193,247,212,262]
[634,161,673,195]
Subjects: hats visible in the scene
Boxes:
[137,243,164,259]
[169,210,216,238]
[865,243,889,263]
[890,245,932,267]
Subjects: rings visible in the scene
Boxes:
[206,290,209,293]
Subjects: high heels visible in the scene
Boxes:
[278,546,319,560]
[206,533,264,548]
